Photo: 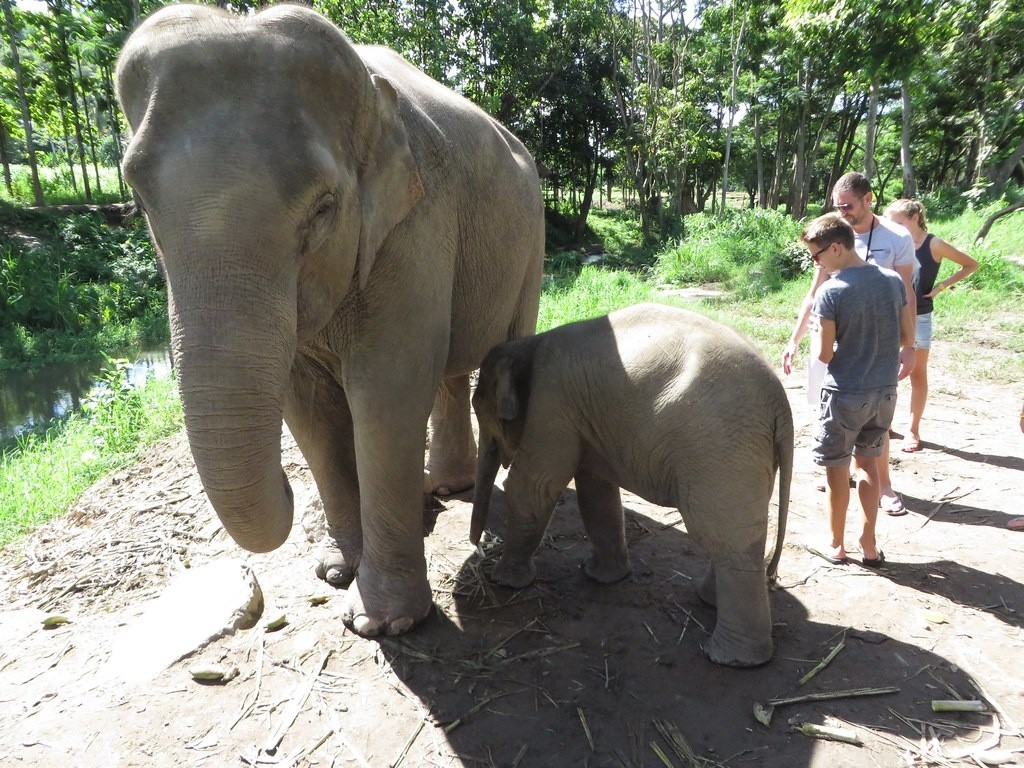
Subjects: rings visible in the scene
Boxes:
[910,370,913,373]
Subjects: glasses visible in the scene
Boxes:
[812,241,841,263]
[832,192,866,211]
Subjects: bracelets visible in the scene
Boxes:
[933,283,946,293]
[1020,413,1024,418]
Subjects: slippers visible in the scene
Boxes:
[878,495,906,516]
[804,540,847,565]
[901,434,921,452]
[889,429,893,434]
[817,475,852,492]
[863,547,884,566]
[1008,518,1024,529]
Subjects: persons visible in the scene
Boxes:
[782,172,916,567]
[883,198,980,452]
[1007,400,1024,530]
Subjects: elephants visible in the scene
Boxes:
[470,301,794,665]
[117,4,545,637]
